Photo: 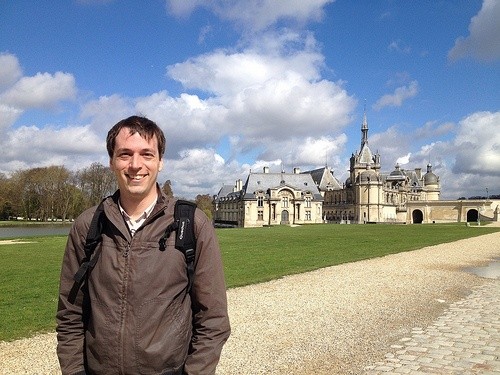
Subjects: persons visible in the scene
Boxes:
[56,114,232,375]
